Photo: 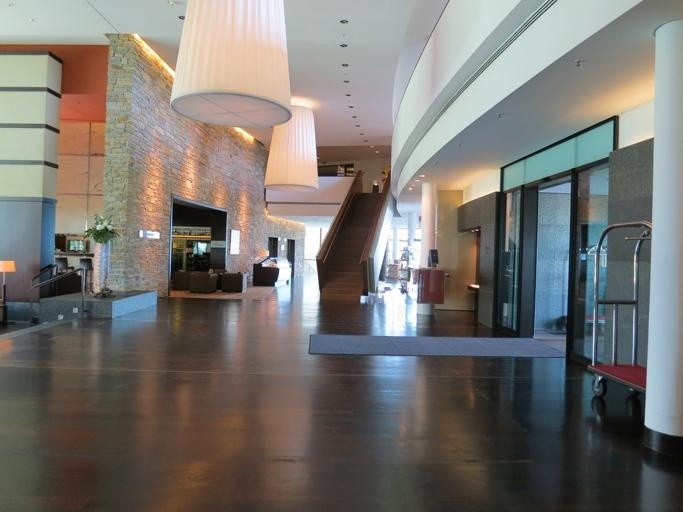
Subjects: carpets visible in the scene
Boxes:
[169,287,275,301]
[306,333,565,358]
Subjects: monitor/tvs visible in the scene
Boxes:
[428,249,438,264]
[69,240,86,251]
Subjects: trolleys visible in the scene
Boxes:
[585,220,652,399]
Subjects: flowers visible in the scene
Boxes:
[82,213,121,244]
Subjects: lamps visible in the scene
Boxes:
[167,0,320,195]
[0,260,16,329]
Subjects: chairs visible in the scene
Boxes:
[173,270,244,293]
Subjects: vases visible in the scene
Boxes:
[91,240,110,295]
